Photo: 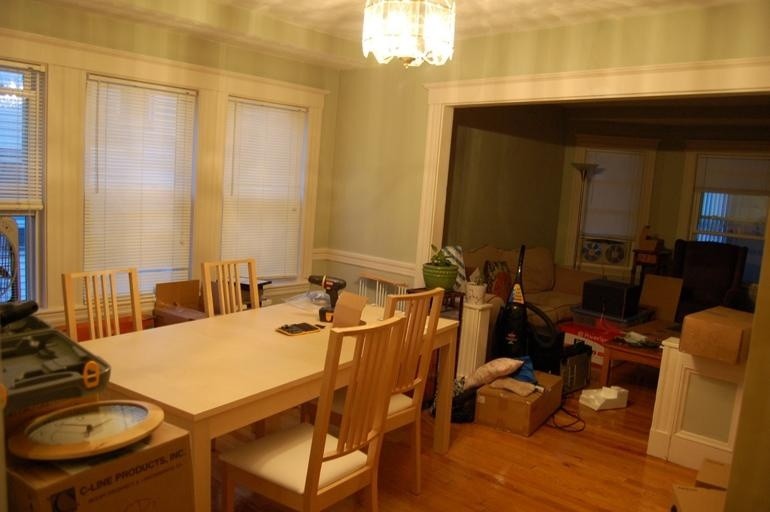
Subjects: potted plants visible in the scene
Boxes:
[423,245,458,291]
[466,279,488,305]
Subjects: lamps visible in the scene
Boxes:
[362,0,456,69]
[570,162,598,272]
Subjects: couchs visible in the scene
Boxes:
[461,243,606,331]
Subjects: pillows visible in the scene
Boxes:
[485,260,512,305]
[555,342,592,395]
[465,357,523,390]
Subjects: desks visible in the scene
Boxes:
[558,304,658,369]
[76,292,460,512]
[407,287,465,408]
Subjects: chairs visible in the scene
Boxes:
[300,288,445,494]
[61,266,143,342]
[219,313,407,511]
[201,256,306,441]
[669,240,748,322]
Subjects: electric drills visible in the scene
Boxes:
[304,275,346,322]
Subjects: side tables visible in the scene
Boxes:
[625,248,671,317]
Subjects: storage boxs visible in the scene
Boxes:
[669,459,732,511]
[8,422,195,512]
[679,306,754,367]
[474,369,563,436]
[638,226,663,250]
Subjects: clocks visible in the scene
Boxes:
[6,398,165,459]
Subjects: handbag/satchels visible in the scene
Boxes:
[431,390,475,423]
